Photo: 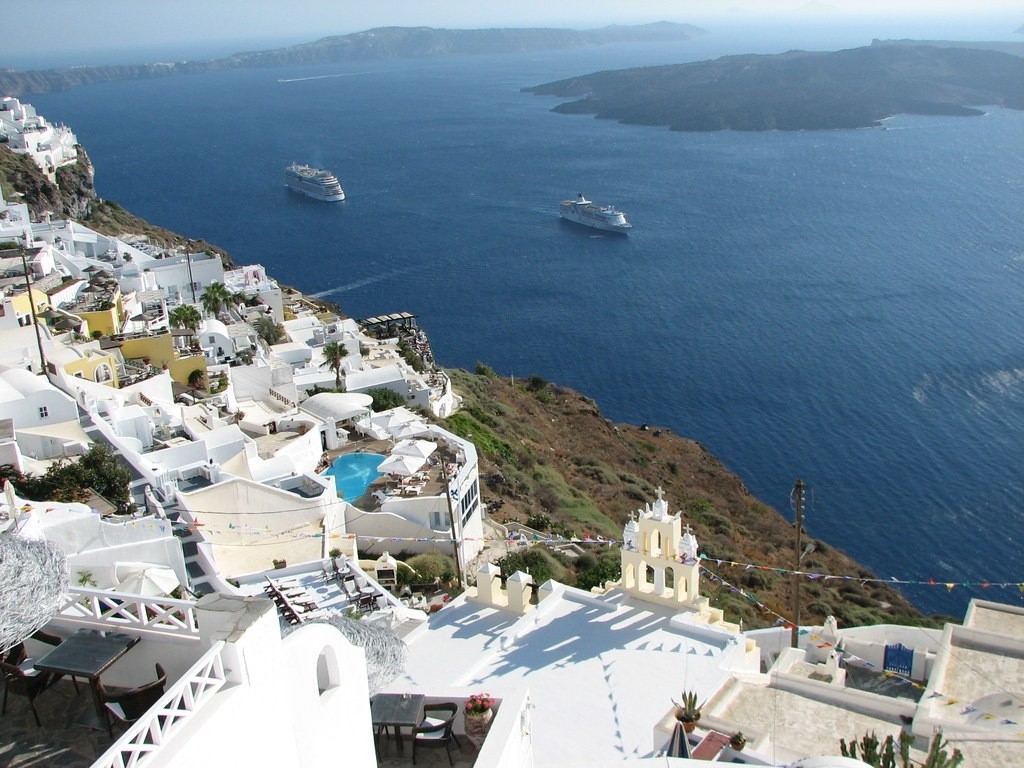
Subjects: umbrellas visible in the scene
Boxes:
[117,567,179,598]
[56,318,81,329]
[130,314,155,321]
[0,533,71,656]
[36,310,62,319]
[372,410,437,490]
[82,266,111,306]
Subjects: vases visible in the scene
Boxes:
[463,708,494,748]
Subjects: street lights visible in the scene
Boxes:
[791,478,806,648]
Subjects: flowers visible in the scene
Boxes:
[465,693,496,715]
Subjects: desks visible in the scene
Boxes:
[338,567,350,577]
[34,627,141,731]
[362,587,375,595]
[371,694,425,751]
[65,286,114,311]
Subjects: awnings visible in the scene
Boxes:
[171,328,195,337]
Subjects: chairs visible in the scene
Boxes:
[264,575,318,625]
[412,702,461,766]
[322,557,351,584]
[0,627,81,727]
[370,471,430,505]
[343,576,383,612]
[97,662,167,736]
[442,462,459,480]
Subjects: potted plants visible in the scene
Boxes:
[273,559,287,569]
[671,689,708,732]
[161,359,169,369]
[142,356,151,365]
[730,731,747,751]
[92,331,103,339]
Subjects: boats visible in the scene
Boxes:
[285,160,345,202]
[559,193,633,233]
